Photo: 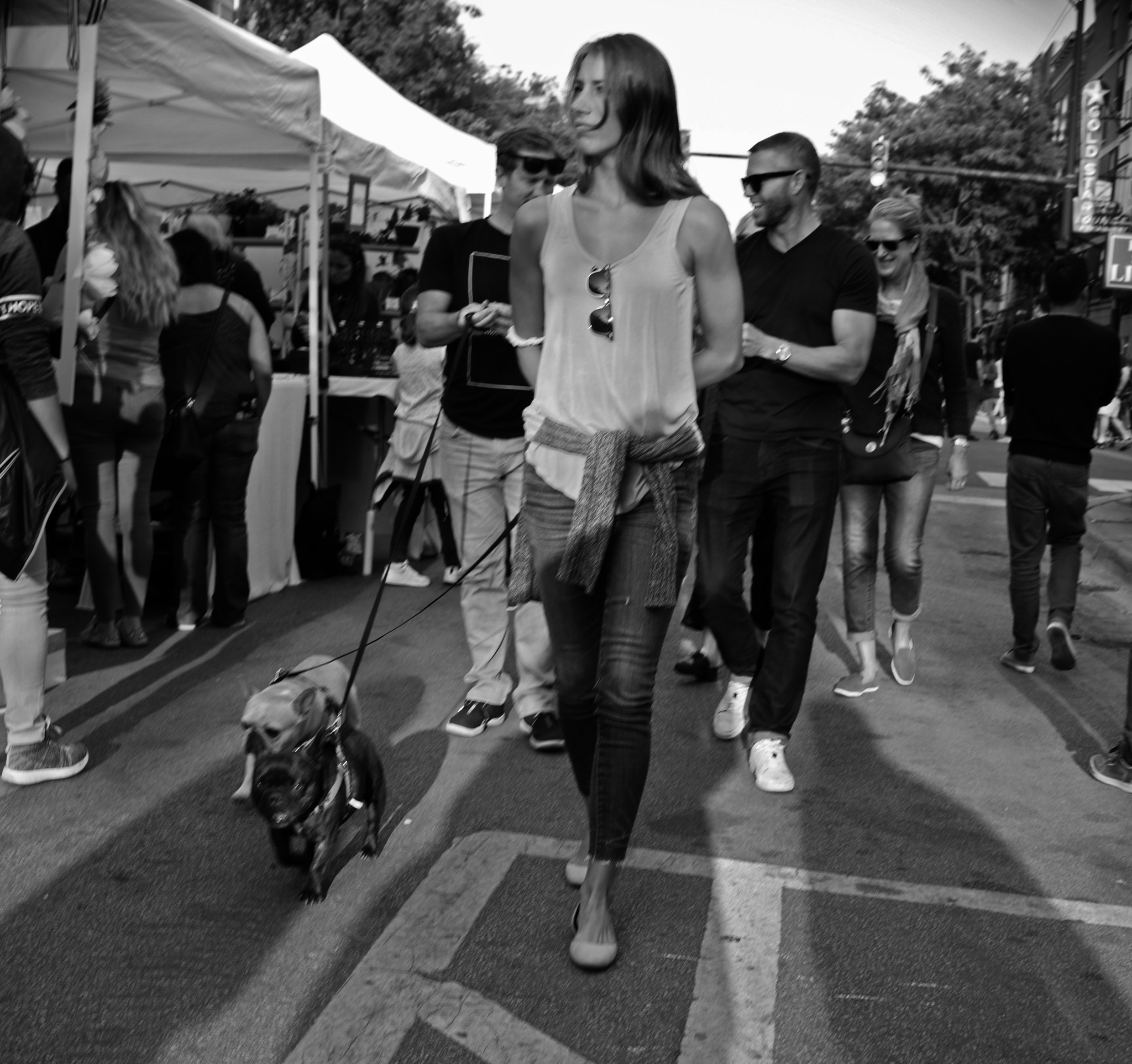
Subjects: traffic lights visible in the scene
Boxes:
[869,138,890,190]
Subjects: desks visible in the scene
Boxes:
[270,367,408,579]
[73,372,308,617]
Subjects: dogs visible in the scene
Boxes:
[252,719,383,906]
[229,655,363,807]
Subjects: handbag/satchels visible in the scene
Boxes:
[149,406,206,491]
[841,421,917,484]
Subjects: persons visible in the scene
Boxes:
[415,35,1132,966]
[0,124,462,786]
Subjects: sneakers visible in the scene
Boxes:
[749,738,795,791]
[446,700,507,736]
[2,714,88,786]
[382,560,430,587]
[713,682,754,738]
[442,566,461,583]
[520,712,565,746]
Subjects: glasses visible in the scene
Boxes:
[509,152,566,176]
[863,235,910,252]
[741,170,811,194]
[584,264,615,339]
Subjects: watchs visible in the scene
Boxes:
[775,338,793,365]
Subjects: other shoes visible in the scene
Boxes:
[834,672,878,698]
[1046,616,1075,670]
[564,841,590,885]
[1000,649,1034,673]
[570,902,619,967]
[82,624,120,647]
[888,620,917,685]
[123,626,148,646]
[1090,751,1132,793]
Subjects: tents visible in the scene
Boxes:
[0,0,569,618]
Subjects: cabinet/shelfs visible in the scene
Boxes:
[227,225,424,328]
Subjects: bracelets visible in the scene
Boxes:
[56,455,70,464]
